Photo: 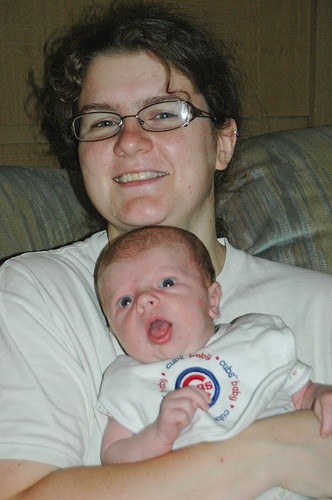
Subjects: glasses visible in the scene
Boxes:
[68,99,213,143]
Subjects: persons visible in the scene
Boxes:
[91,223,332,500]
[1,1,332,500]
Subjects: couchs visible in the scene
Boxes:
[0,122,332,279]
